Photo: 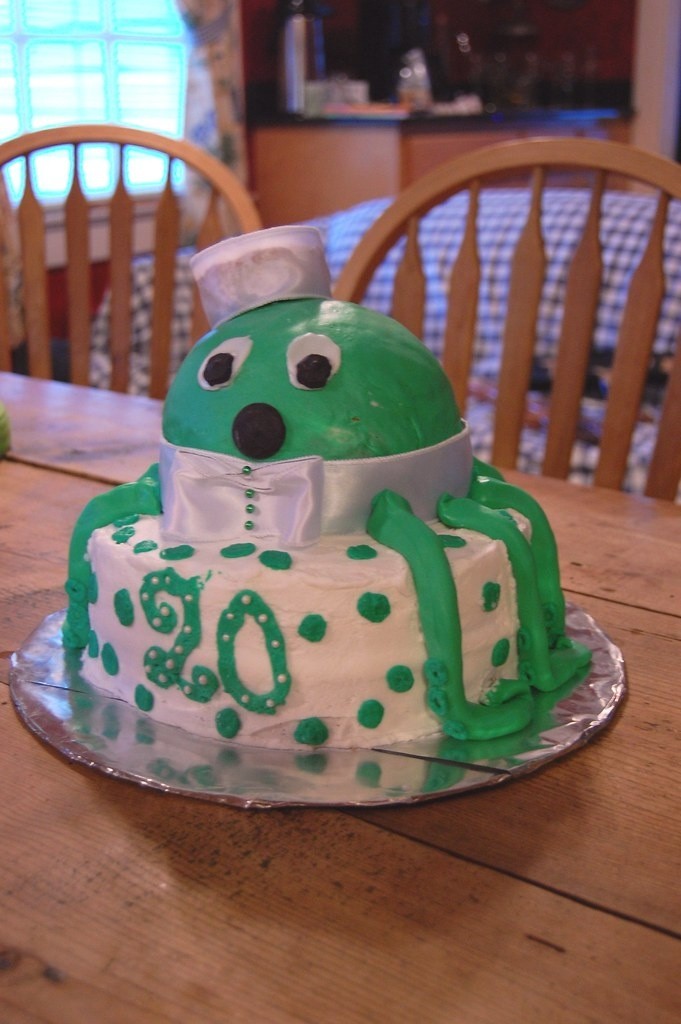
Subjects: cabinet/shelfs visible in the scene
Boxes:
[240,0,681,230]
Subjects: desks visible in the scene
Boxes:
[0,373,681,1024]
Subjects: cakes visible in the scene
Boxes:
[60,224,591,751]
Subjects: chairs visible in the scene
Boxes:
[0,125,260,400]
[329,135,681,505]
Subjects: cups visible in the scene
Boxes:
[304,82,368,115]
